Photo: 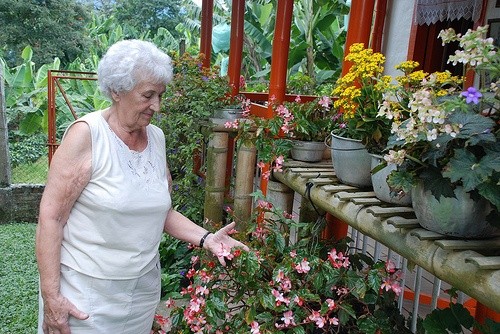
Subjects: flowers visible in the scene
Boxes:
[153,194,410,333]
[222,21,500,226]
[154,50,251,185]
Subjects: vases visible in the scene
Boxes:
[410,176,500,238]
[290,140,328,163]
[369,154,412,206]
[223,109,241,119]
[325,130,373,189]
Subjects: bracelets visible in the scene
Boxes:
[199,231,213,248]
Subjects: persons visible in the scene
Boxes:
[36,39,250,334]
